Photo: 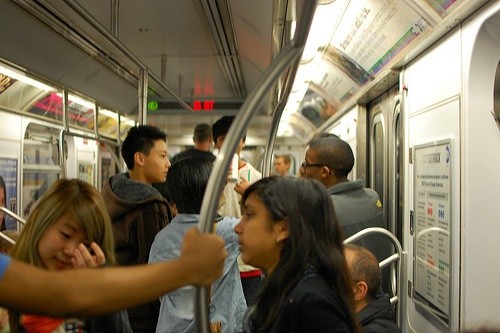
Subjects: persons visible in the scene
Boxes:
[340,244,400,333]
[234,175,356,333]
[0,174,225,333]
[100,125,176,333]
[292,131,393,322]
[149,156,248,333]
[211,114,263,272]
[168,122,215,159]
[9,178,133,333]
[272,153,293,176]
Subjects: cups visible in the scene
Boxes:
[226,153,239,183]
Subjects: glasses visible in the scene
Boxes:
[302,161,328,168]
